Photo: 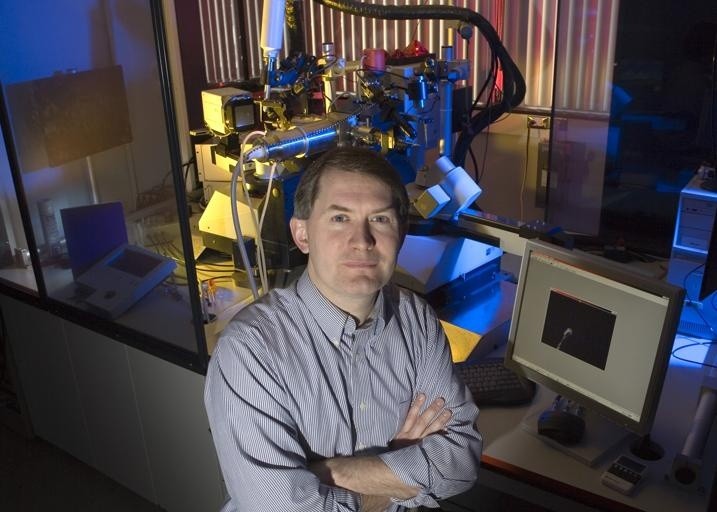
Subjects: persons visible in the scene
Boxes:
[204,147,483,512]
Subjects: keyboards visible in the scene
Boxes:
[454,357,537,408]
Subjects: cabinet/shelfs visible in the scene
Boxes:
[1,295,234,512]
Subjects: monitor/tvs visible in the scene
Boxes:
[502,237,686,464]
